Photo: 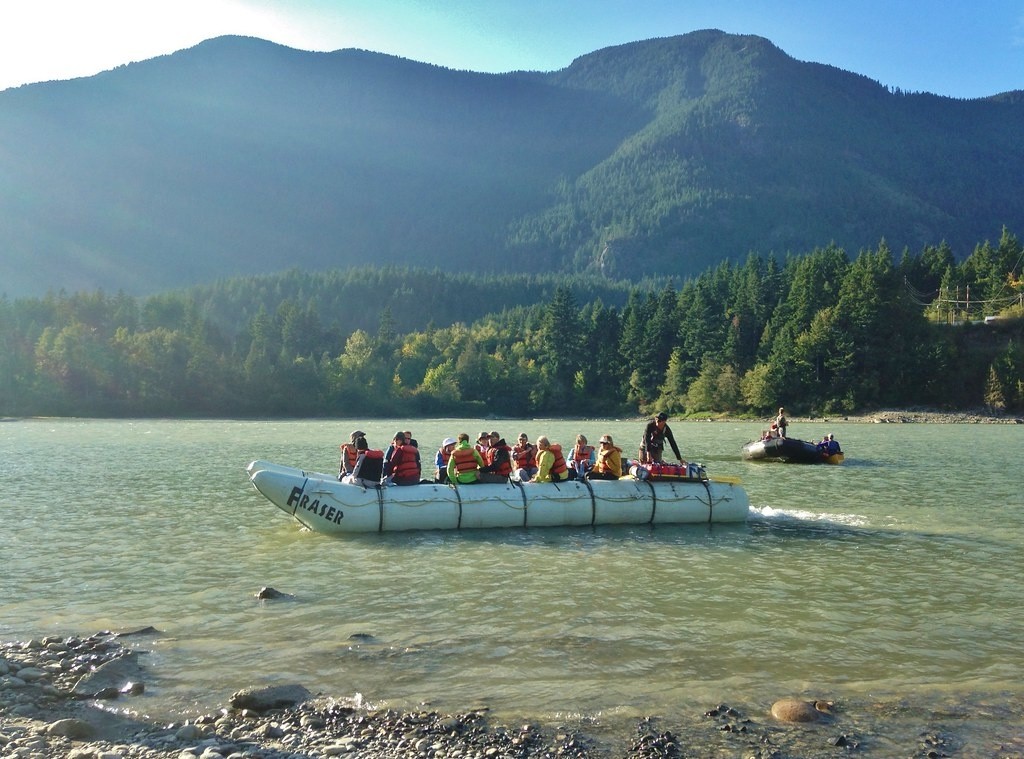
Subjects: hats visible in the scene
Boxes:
[519,433,527,439]
[487,431,499,438]
[599,435,613,443]
[443,438,457,449]
[393,432,405,441]
[350,430,366,445]
[779,407,785,411]
[479,432,488,438]
[655,412,667,420]
[355,437,368,449]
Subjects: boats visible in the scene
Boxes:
[741,436,823,463]
[827,453,844,466]
[243,458,751,536]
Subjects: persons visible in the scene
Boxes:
[338,430,422,488]
[638,412,686,465]
[776,407,789,437]
[434,431,622,484]
[764,431,773,440]
[818,433,841,455]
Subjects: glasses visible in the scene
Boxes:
[655,418,661,422]
[405,437,410,440]
